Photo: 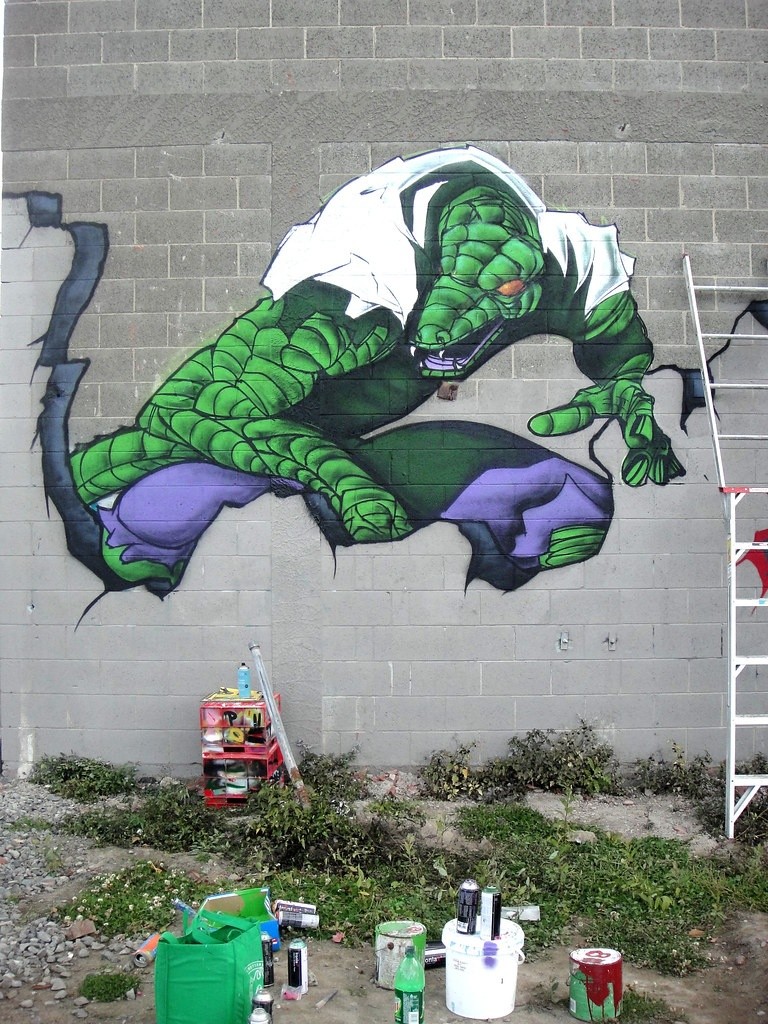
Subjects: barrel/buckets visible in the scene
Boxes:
[441,915,526,1019]
[373,920,427,989]
[566,947,625,1022]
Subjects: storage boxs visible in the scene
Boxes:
[200,691,280,808]
[200,887,280,952]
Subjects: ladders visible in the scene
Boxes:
[679,254,768,842]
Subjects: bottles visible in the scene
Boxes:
[394,946,426,1024]
[480,883,502,940]
[238,663,251,700]
[252,989,275,1017]
[289,938,308,995]
[273,911,319,929]
[261,930,275,988]
[271,899,316,914]
[455,879,481,934]
[248,1007,272,1024]
